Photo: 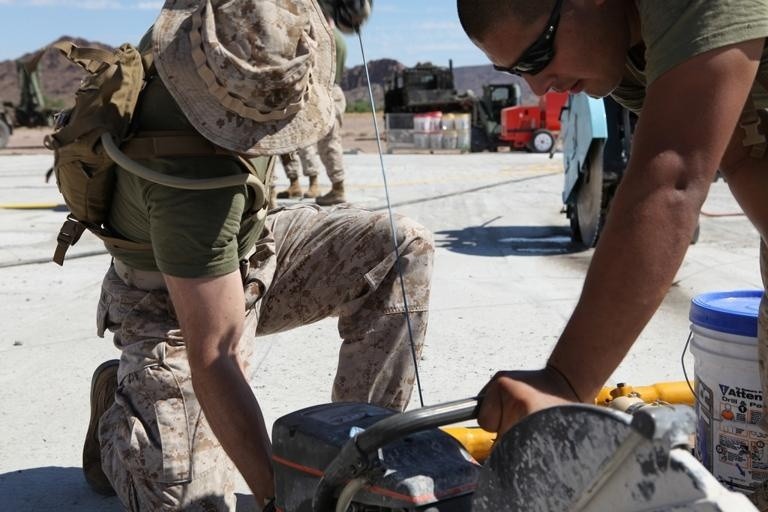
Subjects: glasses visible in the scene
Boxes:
[493,0,563,78]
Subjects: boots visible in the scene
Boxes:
[276,177,302,199]
[316,180,347,206]
[303,176,322,199]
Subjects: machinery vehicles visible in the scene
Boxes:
[502,90,572,154]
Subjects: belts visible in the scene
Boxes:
[111,256,169,292]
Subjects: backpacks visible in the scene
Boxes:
[42,41,270,251]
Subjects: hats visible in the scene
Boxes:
[151,0,338,157]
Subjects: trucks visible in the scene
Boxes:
[376,62,521,154]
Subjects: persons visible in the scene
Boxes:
[278,146,317,200]
[453,0,767,512]
[314,0,351,210]
[80,1,437,512]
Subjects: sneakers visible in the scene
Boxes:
[82,359,121,497]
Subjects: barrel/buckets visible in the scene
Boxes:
[410,110,473,151]
[682,289,766,493]
